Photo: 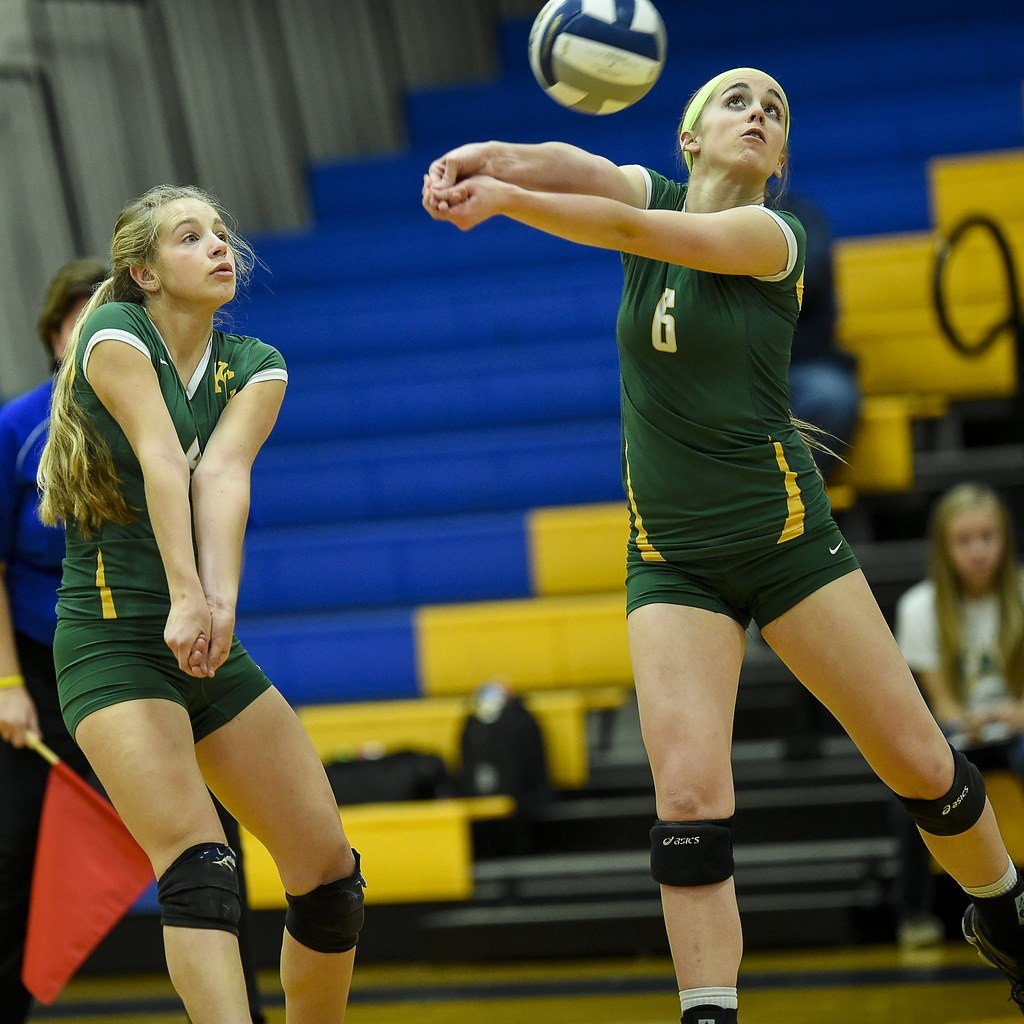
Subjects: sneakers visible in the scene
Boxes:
[960,903,1024,1020]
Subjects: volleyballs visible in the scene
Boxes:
[527,1,668,117]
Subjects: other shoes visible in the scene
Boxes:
[899,919,940,949]
